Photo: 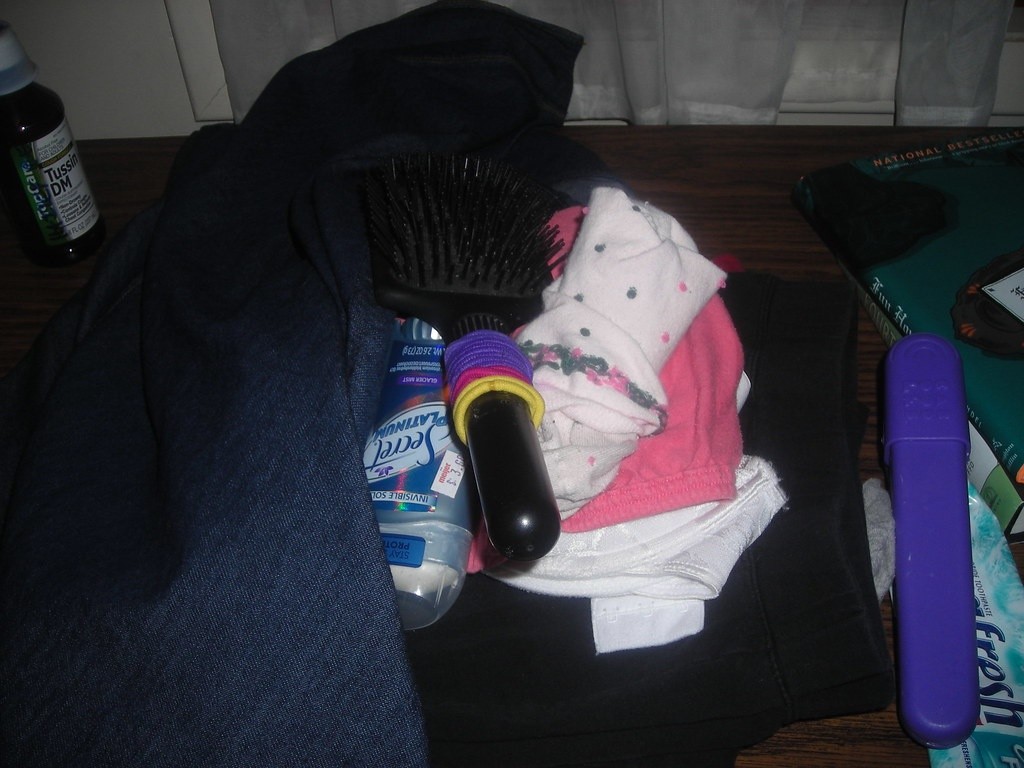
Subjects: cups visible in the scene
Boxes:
[0,28,39,96]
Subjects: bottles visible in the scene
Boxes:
[0,24,106,267]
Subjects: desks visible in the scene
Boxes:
[0,123,1024,768]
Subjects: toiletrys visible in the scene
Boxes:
[2,29,108,251]
[896,480,1022,766]
[361,316,469,631]
